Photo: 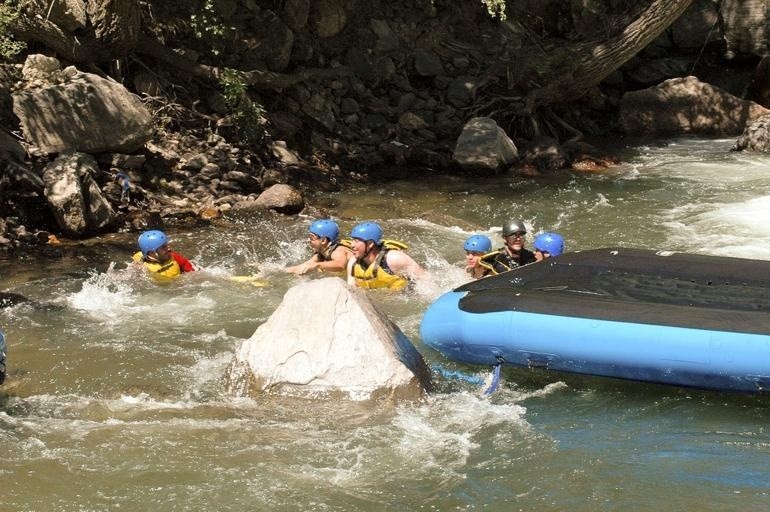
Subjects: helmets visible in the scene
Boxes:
[463,234,492,254]
[138,230,168,257]
[502,218,526,238]
[309,220,340,242]
[350,222,383,247]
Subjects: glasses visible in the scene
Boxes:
[510,233,527,240]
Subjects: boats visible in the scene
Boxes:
[420,233,770,396]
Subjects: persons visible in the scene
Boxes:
[490,219,537,275]
[347,221,436,288]
[282,219,355,276]
[126,230,197,281]
[463,233,491,278]
